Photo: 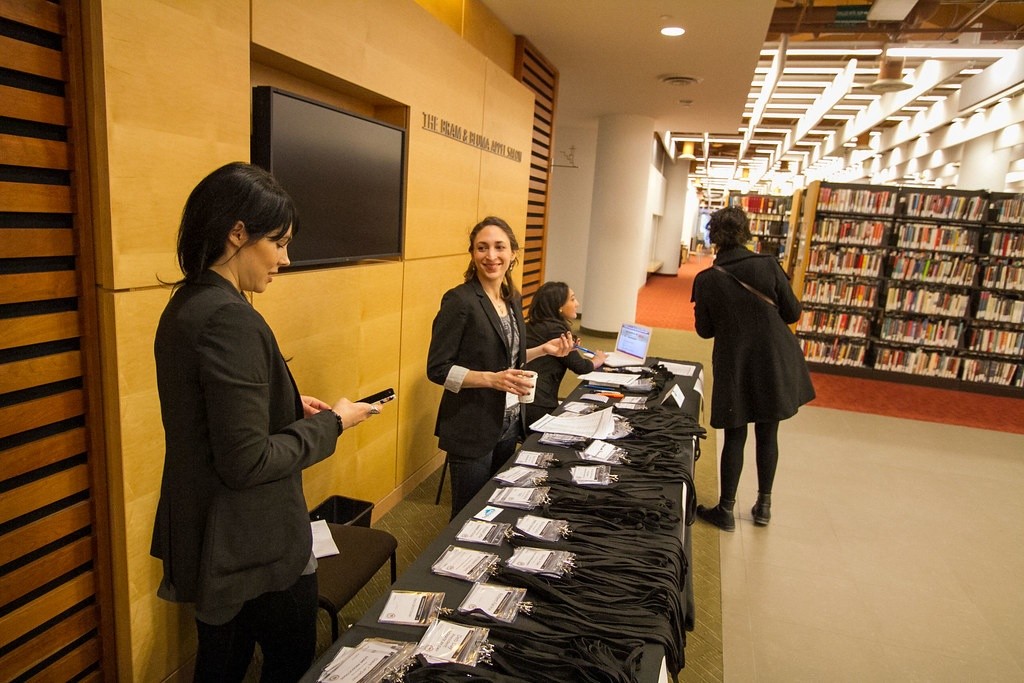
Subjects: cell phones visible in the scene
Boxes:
[356,388,395,405]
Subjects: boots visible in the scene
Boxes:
[751,490,772,525]
[696,496,736,532]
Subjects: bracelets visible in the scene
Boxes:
[328,408,343,437]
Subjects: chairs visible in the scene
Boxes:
[317,521,399,646]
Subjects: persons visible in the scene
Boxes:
[690,207,816,532]
[149,161,397,683]
[426,217,581,526]
[514,281,611,438]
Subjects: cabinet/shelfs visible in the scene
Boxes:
[723,180,1024,399]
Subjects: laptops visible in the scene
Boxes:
[583,321,652,367]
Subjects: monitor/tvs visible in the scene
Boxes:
[252,83,407,273]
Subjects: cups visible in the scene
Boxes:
[516,370,538,404]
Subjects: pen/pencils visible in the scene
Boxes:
[584,385,624,397]
[572,343,595,355]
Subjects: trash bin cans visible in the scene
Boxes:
[309,493,376,527]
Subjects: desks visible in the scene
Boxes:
[298,355,705,683]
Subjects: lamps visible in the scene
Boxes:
[672,40,1024,145]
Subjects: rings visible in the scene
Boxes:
[369,405,380,416]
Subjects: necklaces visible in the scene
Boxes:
[484,290,505,315]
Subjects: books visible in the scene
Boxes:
[747,214,790,235]
[792,186,898,369]
[873,191,987,381]
[962,199,1024,390]
[736,197,792,216]
[742,234,787,268]
